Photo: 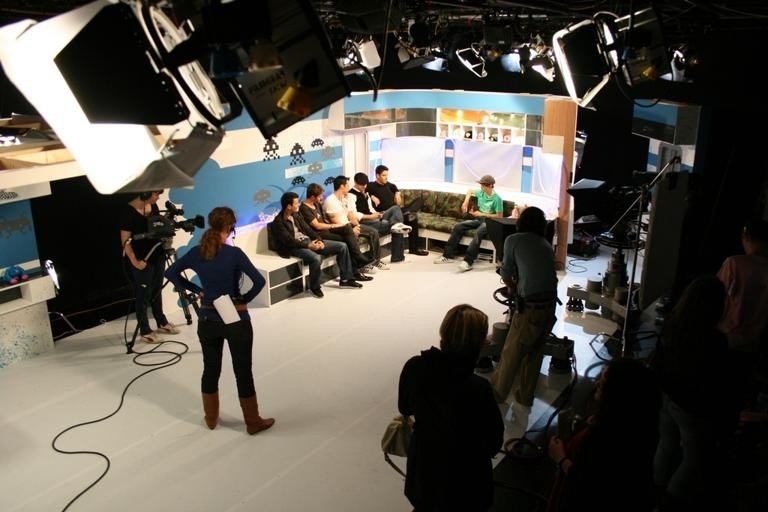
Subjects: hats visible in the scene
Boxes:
[354,173,369,186]
[476,175,495,185]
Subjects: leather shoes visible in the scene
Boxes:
[409,249,430,256]
[404,213,416,222]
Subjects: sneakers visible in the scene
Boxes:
[360,264,377,272]
[390,221,412,234]
[311,286,323,297]
[459,261,474,272]
[433,256,455,264]
[375,261,389,270]
[396,257,412,264]
[339,279,363,288]
[158,323,181,335]
[141,332,163,344]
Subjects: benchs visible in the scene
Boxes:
[230,187,517,308]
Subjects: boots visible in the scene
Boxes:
[239,396,275,435]
[200,390,220,430]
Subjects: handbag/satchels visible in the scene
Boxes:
[380,415,414,459]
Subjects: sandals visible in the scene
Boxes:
[357,273,374,281]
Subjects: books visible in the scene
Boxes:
[212,294,241,326]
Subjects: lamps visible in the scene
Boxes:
[0,0,243,196]
[393,17,625,108]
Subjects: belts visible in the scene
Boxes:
[525,303,555,310]
[200,303,247,312]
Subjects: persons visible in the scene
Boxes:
[164,206,275,436]
[542,223,767,512]
[348,172,413,263]
[324,176,392,275]
[489,207,559,408]
[299,182,374,282]
[272,191,363,298]
[432,175,504,273]
[119,187,181,345]
[365,165,429,256]
[395,303,506,511]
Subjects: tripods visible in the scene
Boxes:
[126,238,200,354]
[589,156,681,359]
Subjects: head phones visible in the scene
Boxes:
[140,191,152,201]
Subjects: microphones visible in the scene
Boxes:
[231,227,236,239]
